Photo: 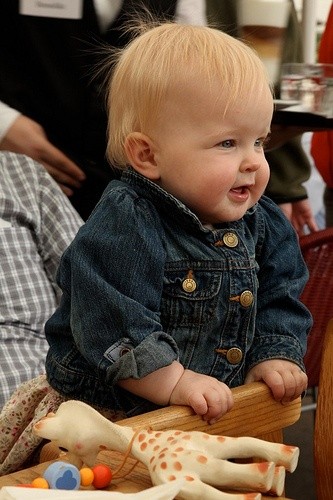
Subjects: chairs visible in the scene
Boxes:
[297,228,333,500]
[0,374,301,500]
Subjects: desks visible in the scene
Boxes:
[272,101,333,130]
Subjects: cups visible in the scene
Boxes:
[280,62,333,112]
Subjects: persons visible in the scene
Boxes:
[43,24,312,424]
[0,0,333,467]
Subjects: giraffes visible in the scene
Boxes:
[34,399,300,500]
